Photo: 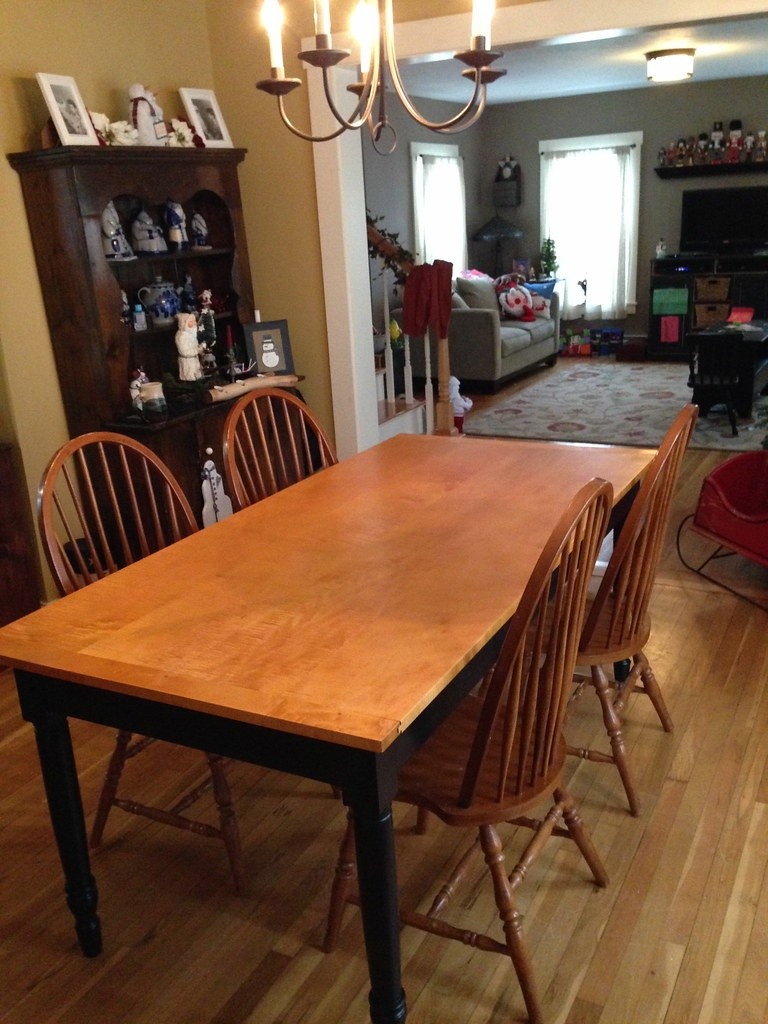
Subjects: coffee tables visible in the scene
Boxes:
[684,319,767,418]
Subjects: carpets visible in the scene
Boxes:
[459,362,768,451]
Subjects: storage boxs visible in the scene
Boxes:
[614,339,647,362]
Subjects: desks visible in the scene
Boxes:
[0,432,658,1024]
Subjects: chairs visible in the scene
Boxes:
[38,431,198,597]
[325,478,613,1024]
[526,405,698,818]
[676,451,768,614]
[222,387,337,511]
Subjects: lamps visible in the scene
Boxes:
[644,47,697,83]
[472,208,524,276]
[256,0,508,155]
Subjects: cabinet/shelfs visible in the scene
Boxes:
[6,144,255,569]
[647,256,768,362]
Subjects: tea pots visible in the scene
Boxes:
[138,274,183,328]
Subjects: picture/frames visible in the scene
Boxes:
[178,87,234,148]
[35,72,101,146]
[242,318,297,376]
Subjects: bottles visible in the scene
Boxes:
[655,238,667,259]
[133,305,148,331]
[140,382,168,423]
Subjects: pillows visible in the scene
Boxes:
[456,277,505,320]
[524,280,556,320]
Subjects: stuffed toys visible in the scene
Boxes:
[449,376,473,436]
[495,281,536,322]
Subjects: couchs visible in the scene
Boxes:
[390,292,559,396]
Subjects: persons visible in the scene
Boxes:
[60,99,85,135]
[175,289,217,381]
[194,103,223,140]
